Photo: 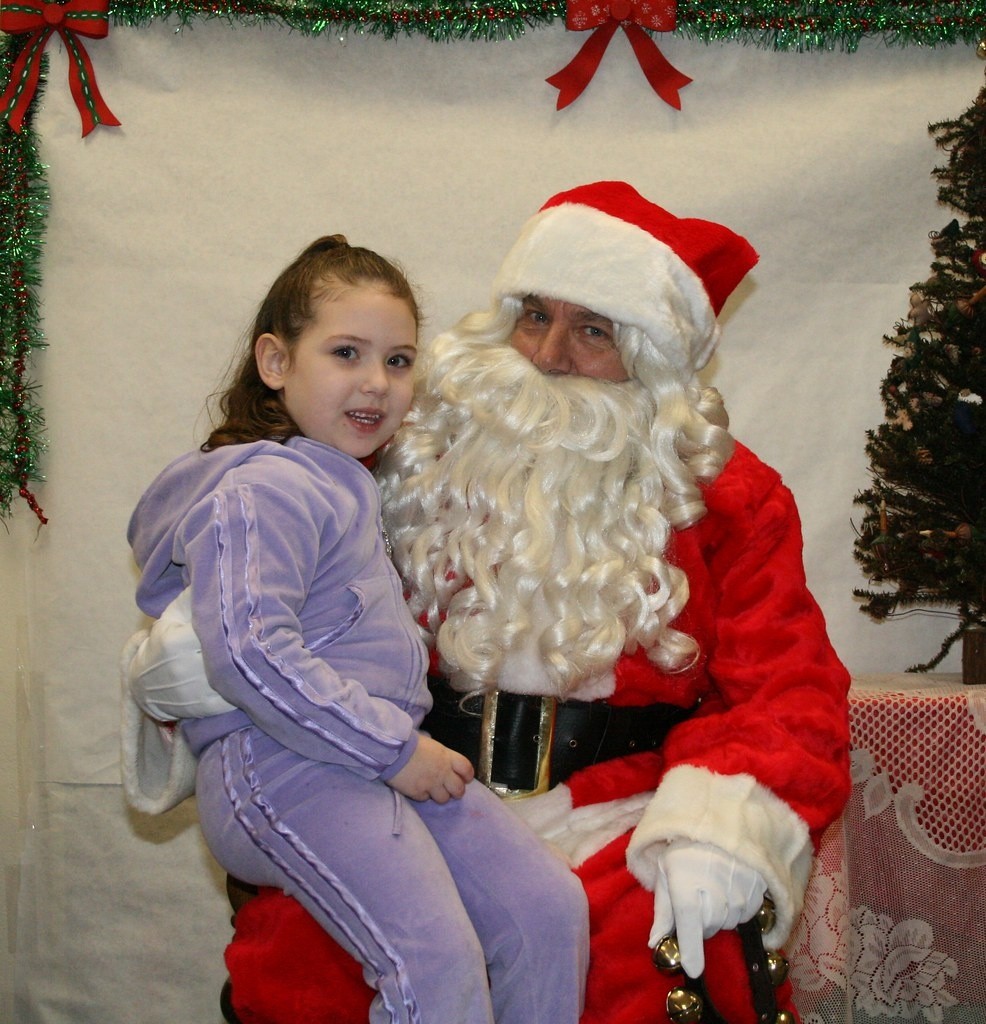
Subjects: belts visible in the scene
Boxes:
[413,665,701,803]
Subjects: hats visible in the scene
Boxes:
[502,180,759,375]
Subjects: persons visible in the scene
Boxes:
[136,232,597,1024]
[118,176,861,1024]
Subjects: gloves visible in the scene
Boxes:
[648,841,769,978]
[126,589,242,723]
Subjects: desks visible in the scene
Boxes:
[797,662,986,1024]
